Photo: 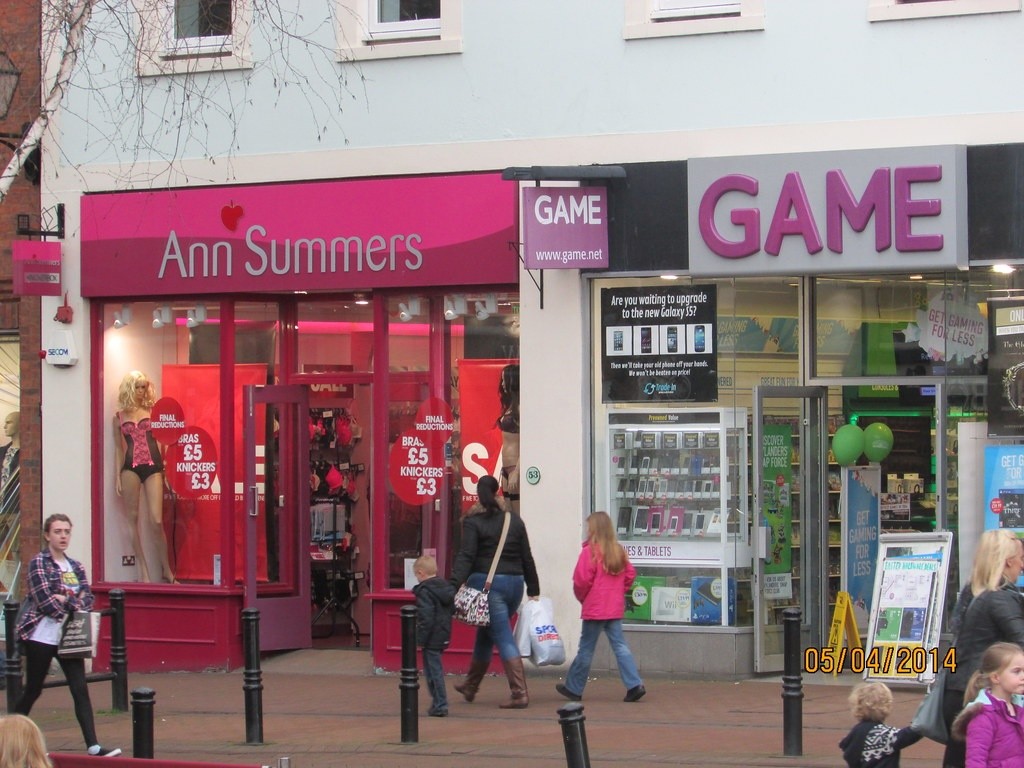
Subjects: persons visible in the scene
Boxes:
[498,365,520,520]
[839,682,925,768]
[556,512,646,702]
[936,528,1024,768]
[411,556,456,717]
[448,475,540,709]
[0,412,23,601]
[112,371,181,584]
[12,514,122,757]
[0,715,51,768]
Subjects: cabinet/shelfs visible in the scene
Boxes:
[612,407,989,638]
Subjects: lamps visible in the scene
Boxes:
[455,296,468,315]
[113,310,125,330]
[186,310,199,328]
[485,294,498,313]
[446,301,458,320]
[160,304,173,323]
[399,303,413,322]
[152,308,166,329]
[474,302,490,321]
[355,291,370,305]
[195,304,208,322]
[408,297,421,316]
[121,302,132,324]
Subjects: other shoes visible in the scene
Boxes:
[428,707,449,716]
[624,684,646,702]
[88,747,123,757]
[555,683,582,702]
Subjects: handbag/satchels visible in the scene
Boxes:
[909,668,950,745]
[58,609,101,659]
[514,595,566,666]
[450,583,491,627]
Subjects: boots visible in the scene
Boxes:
[453,653,489,702]
[499,656,529,708]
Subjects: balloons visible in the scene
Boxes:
[864,422,894,468]
[831,424,865,466]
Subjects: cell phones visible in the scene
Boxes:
[693,325,705,352]
[641,327,652,353]
[613,331,623,351]
[616,453,711,535]
[667,326,677,352]
